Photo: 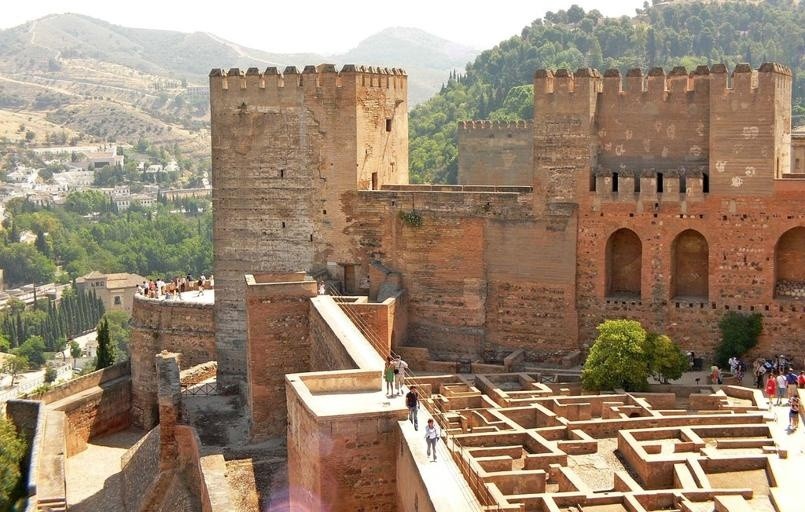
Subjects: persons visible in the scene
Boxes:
[392,355,408,394]
[135,273,215,301]
[750,351,805,432]
[688,351,694,369]
[384,356,395,397]
[405,386,421,432]
[706,354,746,385]
[425,419,440,461]
